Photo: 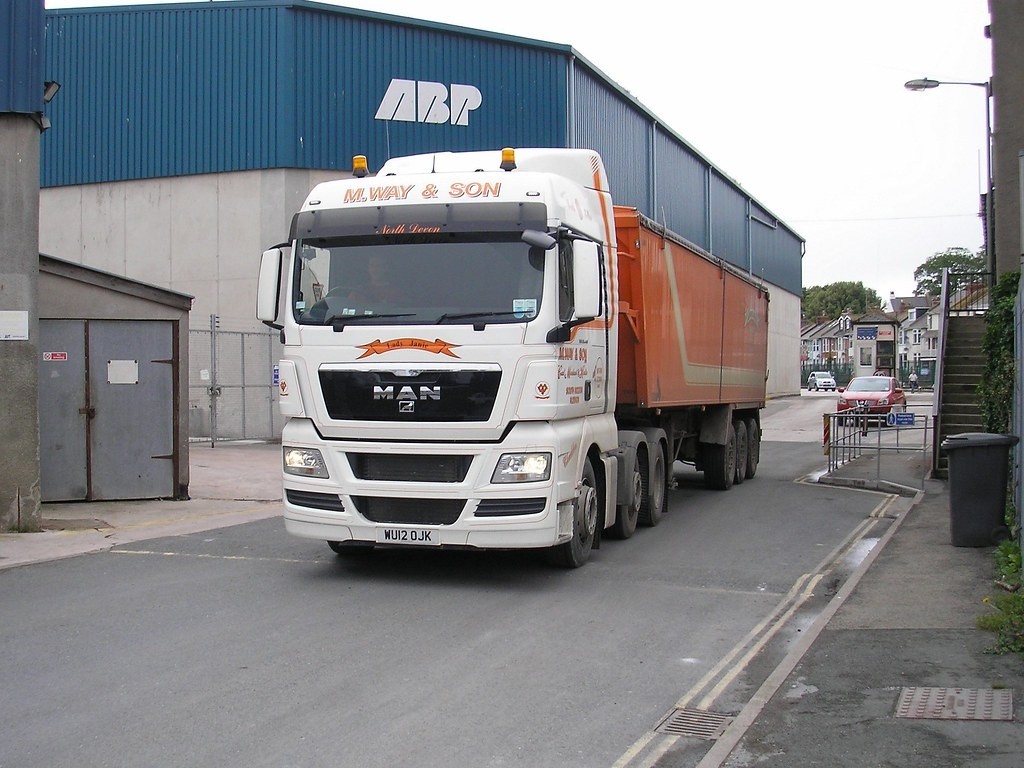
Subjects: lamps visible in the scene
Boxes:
[43,81,61,102]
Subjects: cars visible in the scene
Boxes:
[806,372,836,392]
[836,376,906,427]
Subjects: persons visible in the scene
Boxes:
[345,254,408,306]
[909,370,918,394]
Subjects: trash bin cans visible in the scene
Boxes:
[940,432,1021,547]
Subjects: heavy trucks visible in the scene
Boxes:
[256,147,770,570]
